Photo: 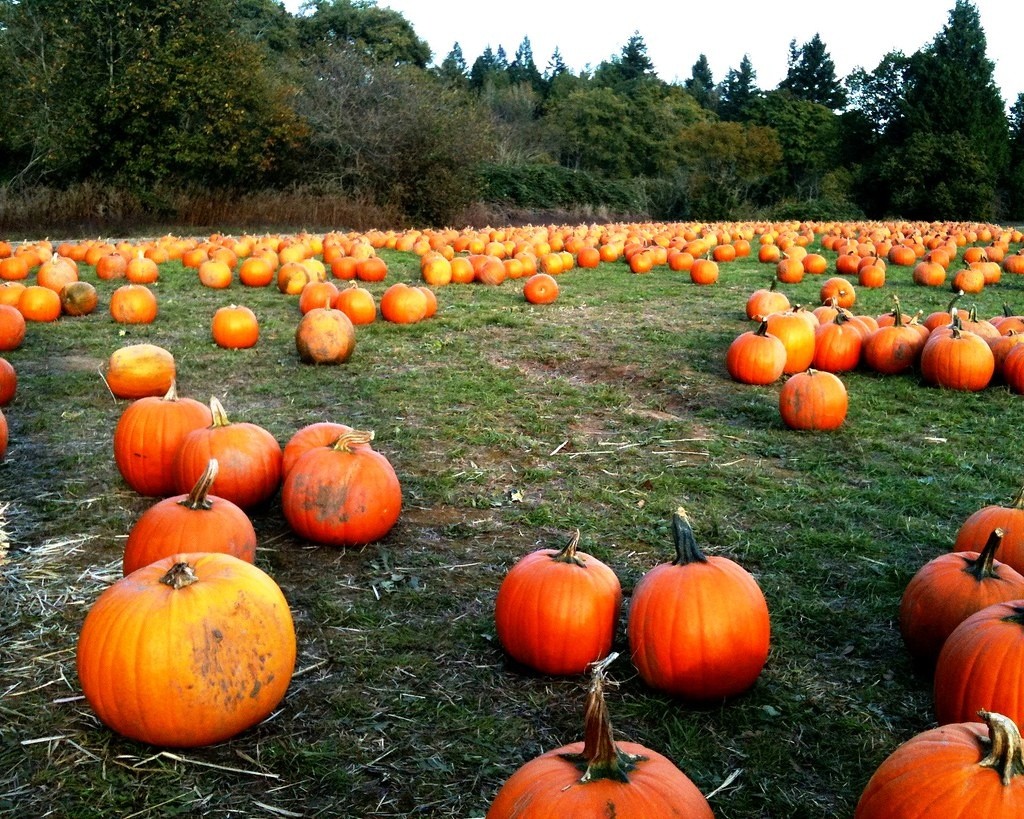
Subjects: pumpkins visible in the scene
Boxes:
[0,220,1024,819]
